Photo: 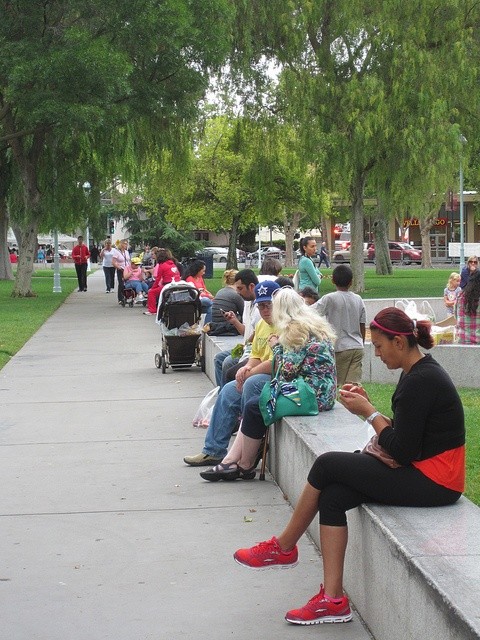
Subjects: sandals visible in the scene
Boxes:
[223,464,257,480]
[199,461,240,481]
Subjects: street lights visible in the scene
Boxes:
[457,133,467,276]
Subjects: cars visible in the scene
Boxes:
[252,247,283,260]
[246,247,265,260]
[203,246,239,263]
[332,242,374,264]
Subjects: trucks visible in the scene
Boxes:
[334,226,351,251]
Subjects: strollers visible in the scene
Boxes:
[154,280,205,374]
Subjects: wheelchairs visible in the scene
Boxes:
[120,266,148,308]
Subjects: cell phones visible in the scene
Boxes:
[218,307,228,317]
[338,387,353,396]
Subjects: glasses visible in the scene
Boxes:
[468,260,478,265]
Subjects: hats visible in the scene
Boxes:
[130,257,143,265]
[252,279,280,307]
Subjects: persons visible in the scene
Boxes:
[185,259,215,327]
[37,245,45,263]
[233,307,465,625]
[143,248,181,316]
[183,280,280,465]
[461,256,479,289]
[317,242,330,269]
[255,258,282,284]
[308,265,366,392]
[90,245,99,264]
[297,237,323,294]
[239,243,247,251]
[124,257,149,303]
[200,287,337,482]
[8,246,18,263]
[213,268,262,391]
[454,269,480,345]
[100,239,116,293]
[112,239,130,304]
[139,245,153,266]
[72,236,90,292]
[227,276,295,436]
[444,273,462,317]
[145,246,184,289]
[297,286,319,305]
[212,268,245,335]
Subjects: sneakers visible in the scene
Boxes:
[136,295,147,303]
[143,311,157,316]
[183,451,223,465]
[231,536,300,569]
[283,582,352,625]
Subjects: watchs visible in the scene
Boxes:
[366,411,381,424]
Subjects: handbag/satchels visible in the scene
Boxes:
[259,349,319,425]
[292,268,300,291]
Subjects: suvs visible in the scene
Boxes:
[50,242,72,257]
[367,241,422,266]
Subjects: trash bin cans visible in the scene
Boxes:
[197,250,214,278]
